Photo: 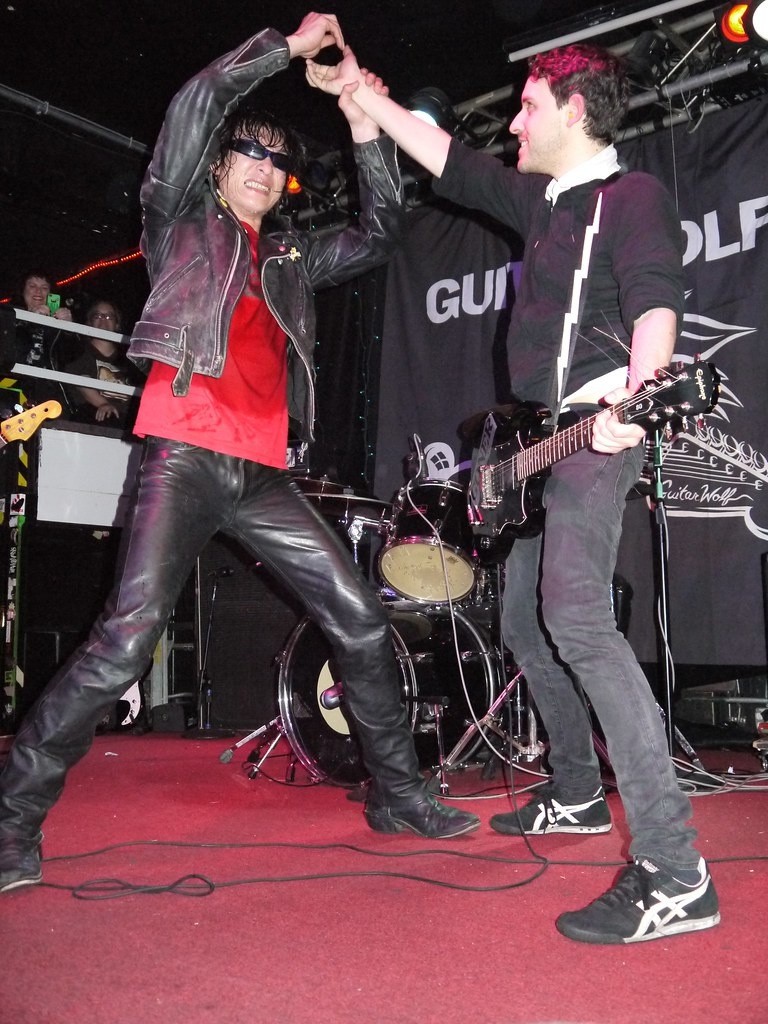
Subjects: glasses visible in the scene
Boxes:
[91,313,115,320]
[225,139,297,173]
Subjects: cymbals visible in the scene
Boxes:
[291,473,336,488]
[302,489,386,504]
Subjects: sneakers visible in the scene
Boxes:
[553,857,720,941]
[493,785,611,833]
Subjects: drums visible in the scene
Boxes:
[279,603,505,789]
[381,481,479,604]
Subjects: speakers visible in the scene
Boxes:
[194,531,310,740]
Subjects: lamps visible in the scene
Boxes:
[712,0,768,55]
[407,85,449,130]
[622,30,666,94]
[285,148,345,196]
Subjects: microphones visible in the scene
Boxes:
[318,685,340,710]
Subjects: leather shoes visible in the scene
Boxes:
[365,793,482,839]
[0,831,43,893]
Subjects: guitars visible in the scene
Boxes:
[466,354,719,567]
[0,400,63,449]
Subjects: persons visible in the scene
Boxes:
[304,44,720,946]
[0,274,84,420]
[63,302,145,430]
[0,13,480,891]
